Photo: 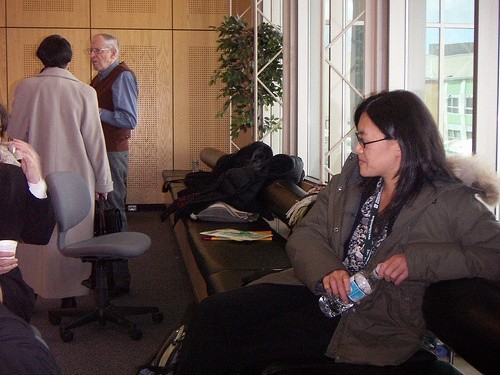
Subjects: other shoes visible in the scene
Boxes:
[61,297,77,323]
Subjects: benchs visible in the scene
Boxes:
[162,148,317,303]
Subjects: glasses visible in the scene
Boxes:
[87,48,110,55]
[355,132,386,149]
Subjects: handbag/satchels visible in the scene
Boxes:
[190,201,259,222]
[81,197,131,295]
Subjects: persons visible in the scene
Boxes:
[172,90,500,375]
[0,104,62,375]
[87,33,139,301]
[6,34,113,309]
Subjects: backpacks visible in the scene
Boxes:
[135,321,187,375]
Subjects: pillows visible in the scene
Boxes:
[187,202,260,224]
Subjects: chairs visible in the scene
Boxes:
[46,171,164,342]
[355,277,500,375]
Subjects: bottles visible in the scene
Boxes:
[318,262,387,317]
